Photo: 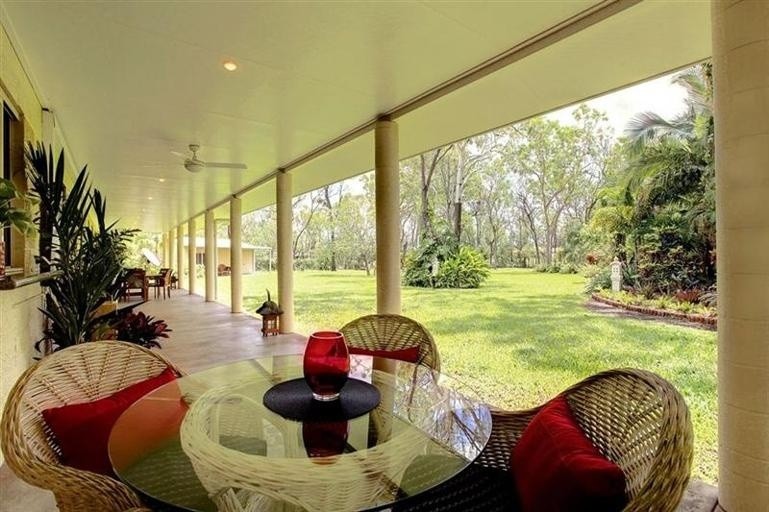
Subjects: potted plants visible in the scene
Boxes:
[0,176,42,289]
[83,228,140,339]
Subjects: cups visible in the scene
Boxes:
[302,420,350,466]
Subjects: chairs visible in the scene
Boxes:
[322,313,442,385]
[0,341,267,512]
[218,264,228,276]
[118,268,172,302]
[391,366,696,512]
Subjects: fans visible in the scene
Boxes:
[143,144,248,173]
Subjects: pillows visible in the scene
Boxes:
[327,342,423,376]
[40,364,191,464]
[508,394,627,512]
